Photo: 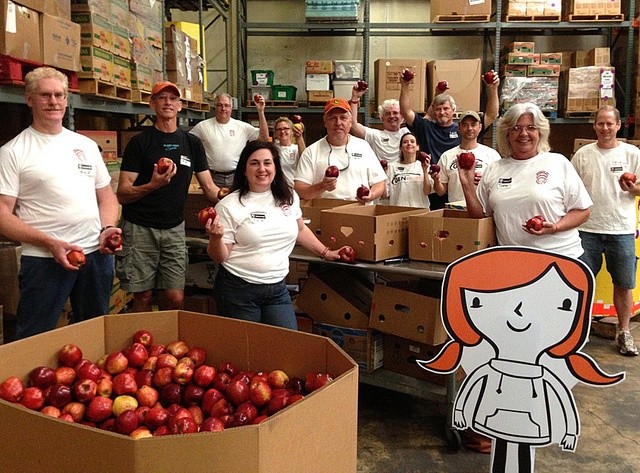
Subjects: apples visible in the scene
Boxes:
[317,231,321,234]
[286,377,305,395]
[143,408,170,430]
[113,373,137,396]
[226,381,250,406]
[484,72,494,83]
[136,370,154,388]
[418,152,429,161]
[218,363,237,376]
[475,173,480,180]
[438,81,446,91]
[457,245,462,250]
[166,341,189,359]
[105,234,123,250]
[260,409,268,415]
[154,368,172,388]
[133,330,153,347]
[291,115,303,123]
[0,376,25,402]
[172,418,197,433]
[620,173,636,186]
[459,153,475,169]
[331,237,336,242]
[129,428,152,439]
[338,247,356,262]
[61,402,85,422]
[121,343,148,367]
[430,164,440,173]
[113,395,139,417]
[66,250,86,267]
[226,412,250,428]
[142,357,158,371]
[305,373,333,392]
[292,124,302,130]
[252,415,268,423]
[272,389,290,398]
[59,344,82,368]
[237,403,258,420]
[167,404,180,414]
[76,362,100,382]
[100,418,116,431]
[75,359,89,379]
[99,368,112,379]
[178,357,195,370]
[187,406,203,424]
[135,407,150,424]
[21,387,45,410]
[527,218,542,231]
[403,71,413,81]
[57,414,74,423]
[97,354,110,369]
[250,380,272,407]
[441,234,448,237]
[160,383,182,406]
[55,366,76,385]
[267,398,287,414]
[254,94,260,103]
[211,399,236,416]
[158,354,177,367]
[287,395,303,404]
[212,373,232,391]
[48,384,72,408]
[40,406,61,418]
[197,207,216,224]
[268,370,289,389]
[105,352,129,374]
[357,187,369,199]
[153,402,163,408]
[173,363,194,384]
[187,347,206,367]
[116,410,139,434]
[28,367,56,390]
[194,365,217,388]
[96,379,113,397]
[168,408,194,427]
[326,166,339,177]
[157,157,173,174]
[153,425,169,435]
[380,160,387,167]
[202,389,228,415]
[218,188,230,198]
[233,371,253,386]
[252,372,269,382]
[86,396,113,422]
[423,244,427,247]
[43,385,53,402]
[123,367,138,373]
[71,379,96,402]
[534,216,545,221]
[359,243,364,246]
[79,421,96,427]
[182,384,205,407]
[199,416,225,432]
[149,344,165,357]
[136,385,159,407]
[358,81,368,90]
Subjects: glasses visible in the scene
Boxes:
[513,124,536,131]
[277,127,290,132]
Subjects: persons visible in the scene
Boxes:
[294,98,388,203]
[191,93,271,188]
[456,102,593,272]
[348,80,447,162]
[427,111,503,203]
[206,140,351,330]
[272,116,307,188]
[570,105,639,356]
[380,133,435,209]
[117,80,225,313]
[397,65,500,166]
[0,65,124,342]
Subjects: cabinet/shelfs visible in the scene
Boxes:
[238,4,367,141]
[498,2,624,125]
[3,85,208,129]
[161,0,236,106]
[367,4,498,149]
[176,236,466,449]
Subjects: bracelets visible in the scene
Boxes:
[99,225,114,231]
[321,247,329,258]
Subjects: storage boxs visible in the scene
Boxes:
[559,48,616,111]
[321,204,430,262]
[111,22,132,60]
[185,191,221,230]
[333,59,362,80]
[307,90,333,102]
[499,77,558,111]
[134,36,165,73]
[0,309,358,473]
[66,278,127,325]
[297,198,365,246]
[306,60,331,74]
[76,130,117,162]
[272,85,297,101]
[110,2,129,30]
[102,159,122,194]
[384,333,466,386]
[71,13,112,52]
[130,65,164,92]
[501,53,540,66]
[305,74,329,91]
[527,65,560,77]
[0,0,39,66]
[430,0,491,22]
[77,46,113,83]
[71,1,110,13]
[295,269,374,330]
[165,27,202,102]
[251,69,274,86]
[332,81,358,102]
[427,59,481,112]
[499,65,527,78]
[540,53,562,65]
[374,60,427,113]
[113,55,132,88]
[502,1,561,16]
[408,208,498,264]
[368,281,449,346]
[506,41,535,53]
[39,12,81,73]
[11,0,71,22]
[563,0,621,15]
[251,84,270,100]
[0,241,23,315]
[574,138,627,153]
[315,321,384,373]
[130,0,163,25]
[306,1,360,26]
[112,1,129,10]
[130,12,165,49]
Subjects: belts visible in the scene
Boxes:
[210,168,236,175]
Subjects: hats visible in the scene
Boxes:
[459,110,481,122]
[324,97,351,115]
[151,80,180,99]
